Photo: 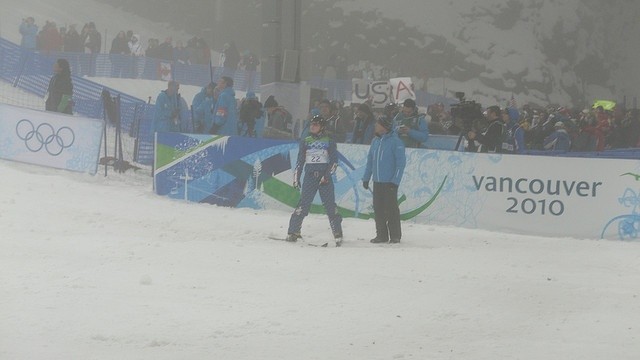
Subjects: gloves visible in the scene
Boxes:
[293,182,300,189]
[389,183,397,191]
[363,182,368,189]
[318,176,331,187]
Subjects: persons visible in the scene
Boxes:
[344,102,375,145]
[385,101,398,119]
[502,104,640,151]
[128,35,144,57]
[285,115,343,248]
[150,79,190,178]
[37,18,102,54]
[44,58,74,115]
[389,98,429,148]
[301,99,335,139]
[126,30,134,41]
[219,43,229,67]
[18,16,38,49]
[145,36,211,65]
[332,101,342,109]
[361,115,406,244]
[224,40,241,70]
[190,74,279,134]
[436,102,449,122]
[312,99,320,108]
[109,30,129,54]
[467,105,502,152]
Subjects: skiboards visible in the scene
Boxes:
[267,238,344,247]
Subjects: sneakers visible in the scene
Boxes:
[370,237,388,243]
[286,234,301,242]
[391,238,400,243]
[334,234,343,247]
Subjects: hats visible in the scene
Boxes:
[358,104,370,111]
[378,114,393,130]
[264,96,277,107]
[403,99,415,106]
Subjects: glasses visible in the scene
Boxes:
[310,121,321,125]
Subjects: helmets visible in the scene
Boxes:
[309,115,327,139]
